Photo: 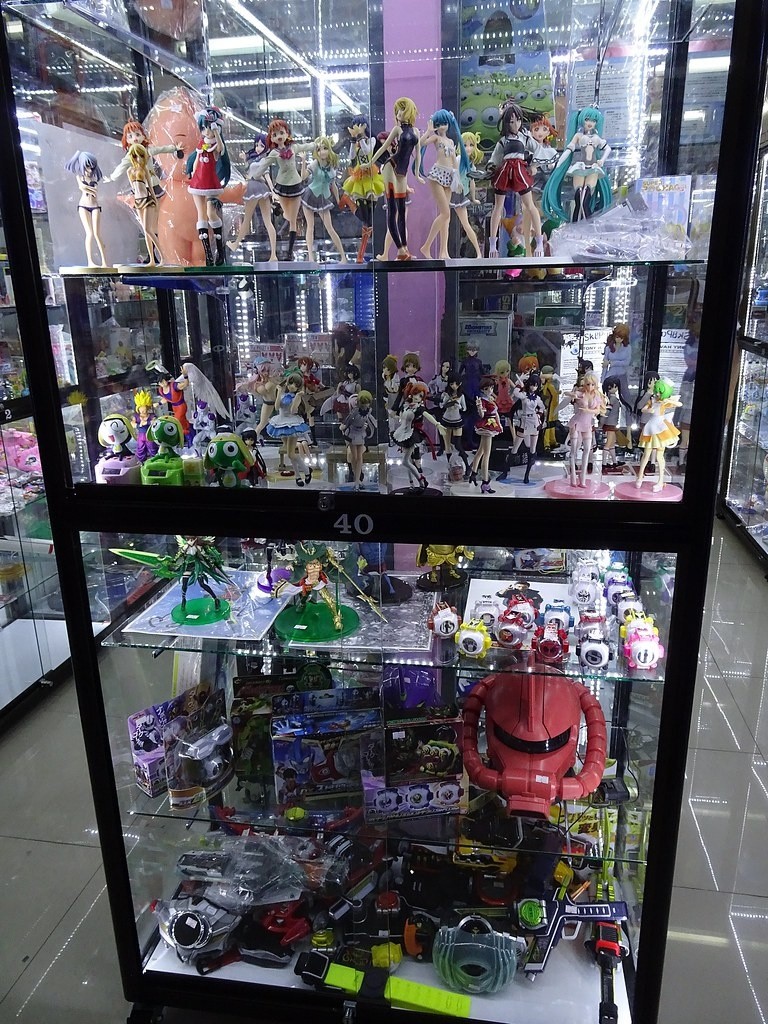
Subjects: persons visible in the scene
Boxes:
[278,769,307,806]
[65,95,683,630]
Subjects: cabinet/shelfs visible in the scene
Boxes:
[0,0,768,1024]
